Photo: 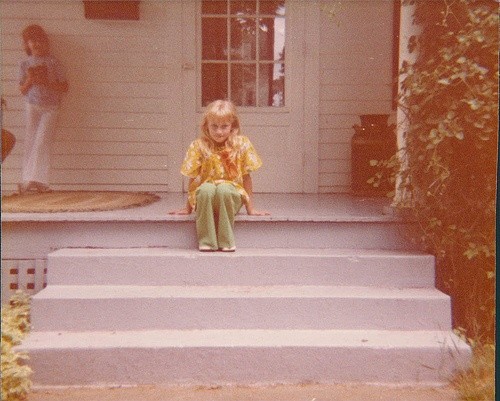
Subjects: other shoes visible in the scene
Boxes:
[199,245,217,251]
[220,246,235,252]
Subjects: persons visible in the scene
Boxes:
[18,24,69,192]
[167,100,272,252]
